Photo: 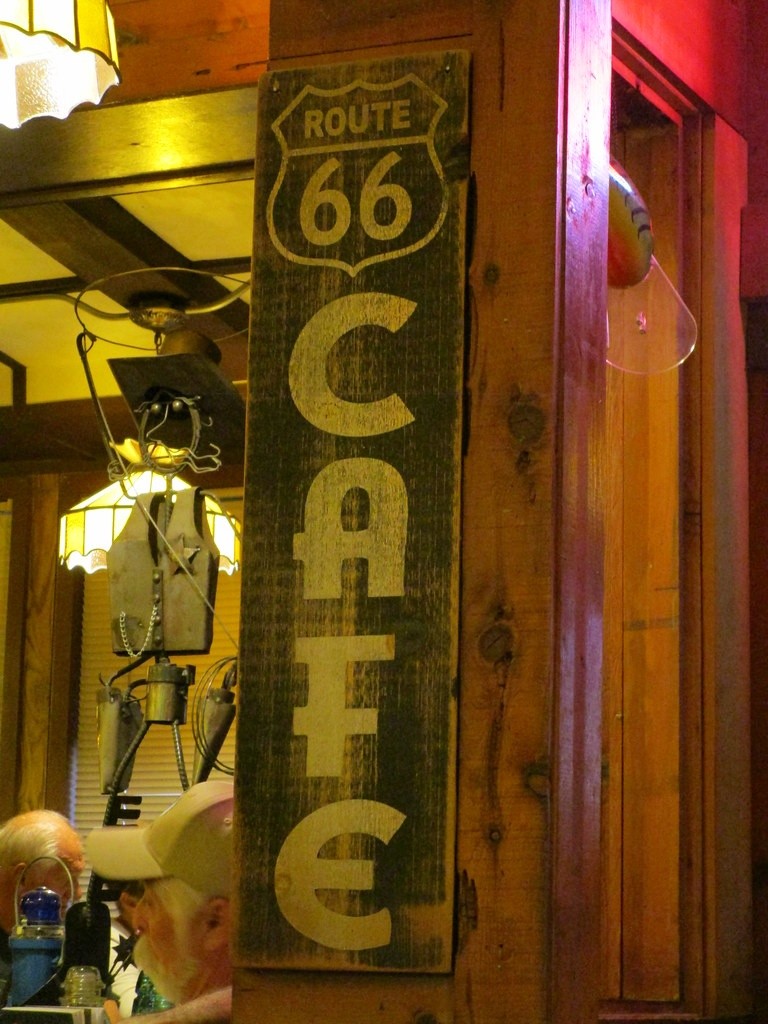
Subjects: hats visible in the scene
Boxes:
[86,778,235,901]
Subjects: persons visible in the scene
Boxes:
[86,779,234,1023]
[96,881,178,1021]
[0,807,82,1023]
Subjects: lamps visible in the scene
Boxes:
[57,438,242,576]
[0,0,122,130]
